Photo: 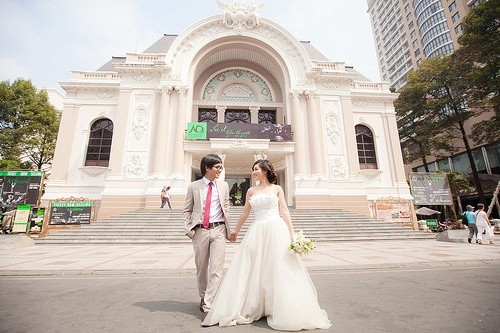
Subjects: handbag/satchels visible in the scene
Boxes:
[485,226,495,239]
[462,212,468,226]
[162,191,165,201]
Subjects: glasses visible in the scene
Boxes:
[212,165,223,170]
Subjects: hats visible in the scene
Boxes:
[466,205,475,208]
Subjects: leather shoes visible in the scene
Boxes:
[202,311,212,326]
[200,299,206,312]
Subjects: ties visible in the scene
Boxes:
[203,181,212,228]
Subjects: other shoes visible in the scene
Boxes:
[468,238,471,243]
[478,240,482,244]
[476,241,478,243]
[490,241,494,244]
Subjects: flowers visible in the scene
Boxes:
[287,231,319,260]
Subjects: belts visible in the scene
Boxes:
[196,222,224,229]
